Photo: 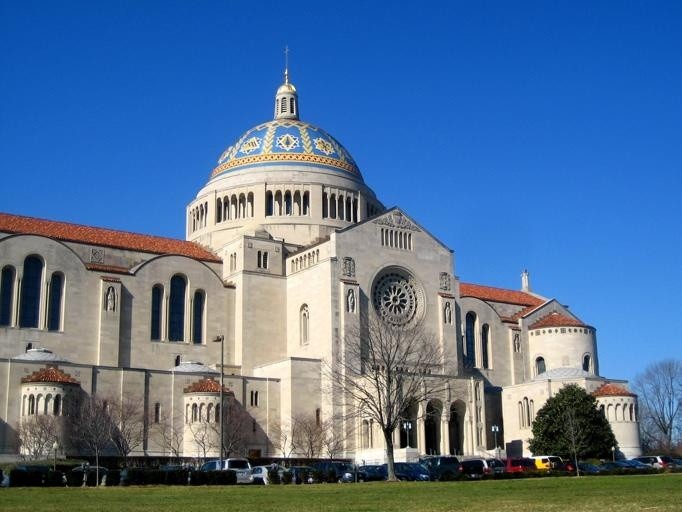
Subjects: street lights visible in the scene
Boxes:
[492,424,499,449]
[53,441,59,472]
[404,419,413,449]
[212,335,224,470]
[611,446,615,463]
